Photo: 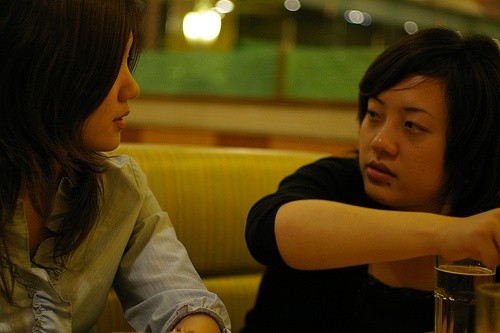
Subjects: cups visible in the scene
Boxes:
[475,284,500,333]
[434,256,496,333]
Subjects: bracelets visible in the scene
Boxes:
[170,327,194,333]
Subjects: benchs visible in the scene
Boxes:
[97,142,333,333]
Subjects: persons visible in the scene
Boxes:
[237,26,500,333]
[0,0,231,333]
